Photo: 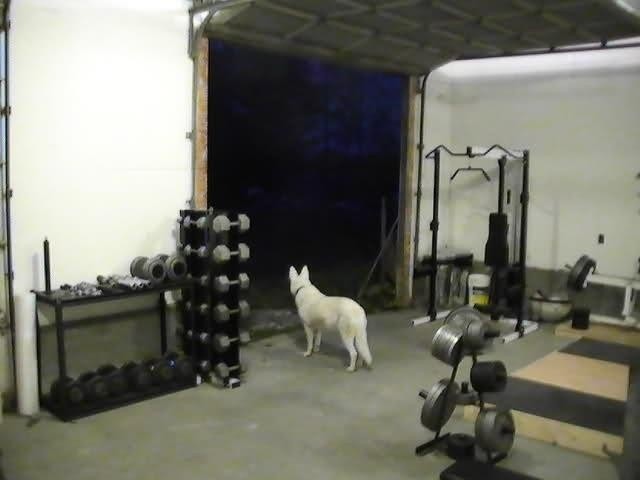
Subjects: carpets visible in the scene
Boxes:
[465,336,639,461]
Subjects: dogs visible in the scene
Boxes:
[289,265,373,373]
